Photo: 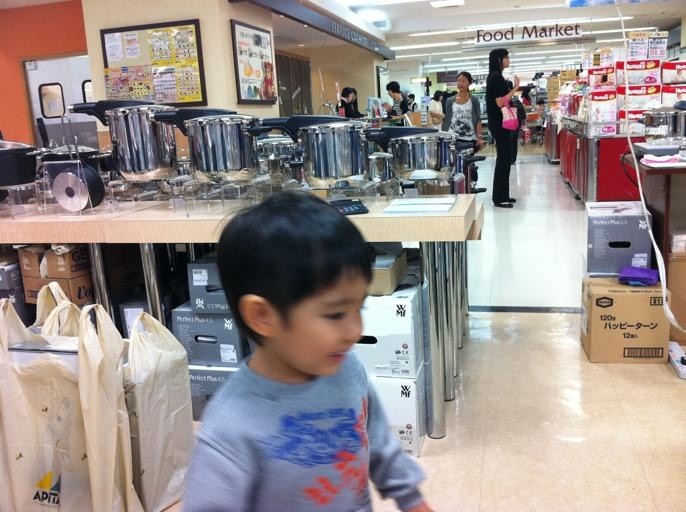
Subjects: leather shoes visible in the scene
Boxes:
[509,198,516,202]
[495,202,513,208]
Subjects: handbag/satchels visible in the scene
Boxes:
[501,97,519,131]
[433,117,442,124]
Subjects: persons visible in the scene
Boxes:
[336,88,362,120]
[484,48,526,209]
[442,72,482,191]
[353,90,367,119]
[386,80,408,126]
[181,191,436,512]
[522,88,532,104]
[532,99,544,133]
[406,93,418,115]
[425,90,446,132]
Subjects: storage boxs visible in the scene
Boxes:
[583,200,653,278]
[349,281,429,459]
[0,243,144,328]
[579,275,672,365]
[170,252,254,422]
[663,251,685,347]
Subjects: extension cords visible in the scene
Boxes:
[668,341,686,380]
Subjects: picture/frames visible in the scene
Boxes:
[229,18,277,104]
[98,18,207,107]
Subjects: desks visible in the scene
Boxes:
[619,154,686,275]
[0,193,485,440]
[451,198,485,377]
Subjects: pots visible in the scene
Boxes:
[1,96,484,212]
[642,106,685,142]
